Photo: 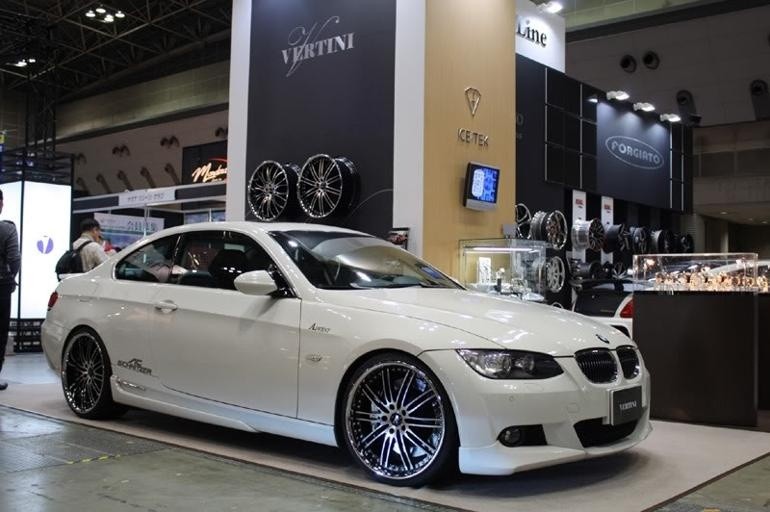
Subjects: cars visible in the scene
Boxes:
[42,222,653,486]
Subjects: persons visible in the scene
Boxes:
[72,217,109,271]
[0,189,22,391]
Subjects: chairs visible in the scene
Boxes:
[208,248,250,290]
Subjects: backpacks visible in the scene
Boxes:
[54,239,94,281]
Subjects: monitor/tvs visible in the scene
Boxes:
[463,161,501,211]
